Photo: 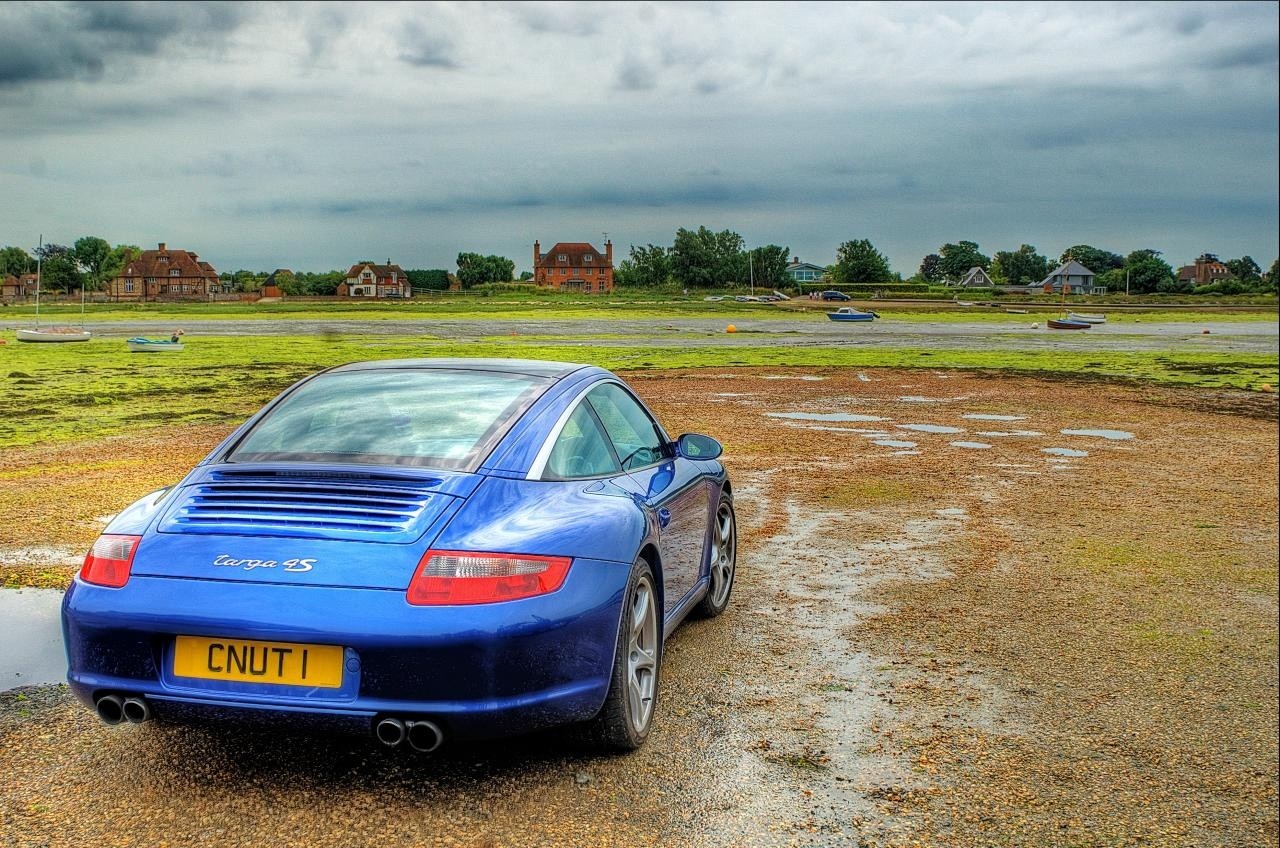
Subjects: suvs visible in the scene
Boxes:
[821,291,851,302]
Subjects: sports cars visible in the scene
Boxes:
[61,355,737,759]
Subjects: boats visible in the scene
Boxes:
[704,250,791,303]
[956,301,973,306]
[1005,307,1029,314]
[1064,308,1106,324]
[125,337,185,352]
[825,307,880,322]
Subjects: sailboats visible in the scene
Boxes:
[15,234,91,342]
[1047,247,1092,329]
